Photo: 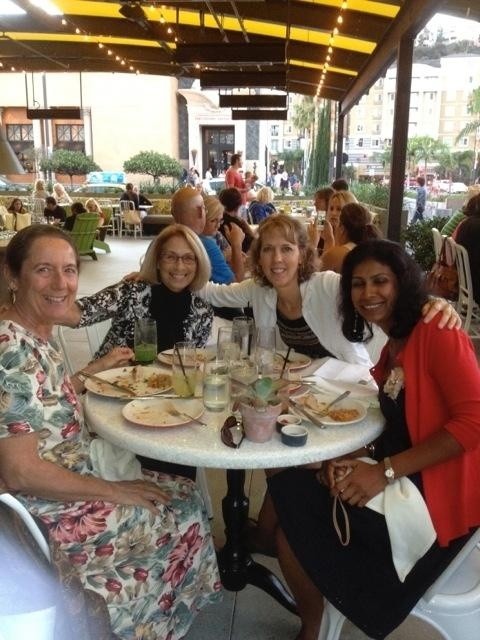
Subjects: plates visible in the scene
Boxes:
[157,347,216,368]
[83,366,173,398]
[288,385,312,401]
[121,394,205,428]
[291,395,367,426]
[272,350,311,370]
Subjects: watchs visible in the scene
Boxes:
[384,457,395,486]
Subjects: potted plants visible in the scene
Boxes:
[233,376,287,443]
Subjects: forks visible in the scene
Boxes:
[317,391,351,418]
[165,403,207,426]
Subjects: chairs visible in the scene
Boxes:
[58,320,114,377]
[1,493,131,640]
[0,196,154,260]
[431,227,480,339]
[318,525,480,639]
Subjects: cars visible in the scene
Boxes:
[0,176,30,193]
[200,178,264,195]
[71,185,123,196]
[438,180,451,192]
[451,182,467,193]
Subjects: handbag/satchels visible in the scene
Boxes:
[423,237,459,302]
[332,457,386,547]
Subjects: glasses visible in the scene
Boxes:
[207,218,224,225]
[221,416,245,448]
[158,253,198,265]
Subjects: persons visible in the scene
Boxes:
[1,224,214,368]
[125,217,463,368]
[0,224,222,638]
[1,151,480,294]
[250,241,480,640]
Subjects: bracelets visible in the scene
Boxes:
[365,444,375,459]
[75,373,85,384]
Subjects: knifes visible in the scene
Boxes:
[288,400,326,429]
[79,370,137,397]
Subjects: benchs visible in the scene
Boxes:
[142,198,316,226]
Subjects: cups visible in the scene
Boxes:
[283,203,292,215]
[48,216,55,225]
[305,206,316,221]
[134,317,157,365]
[202,316,277,412]
[172,342,197,399]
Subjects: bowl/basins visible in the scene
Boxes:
[281,425,308,446]
[276,414,302,433]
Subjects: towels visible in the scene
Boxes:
[338,458,437,582]
[86,422,144,482]
